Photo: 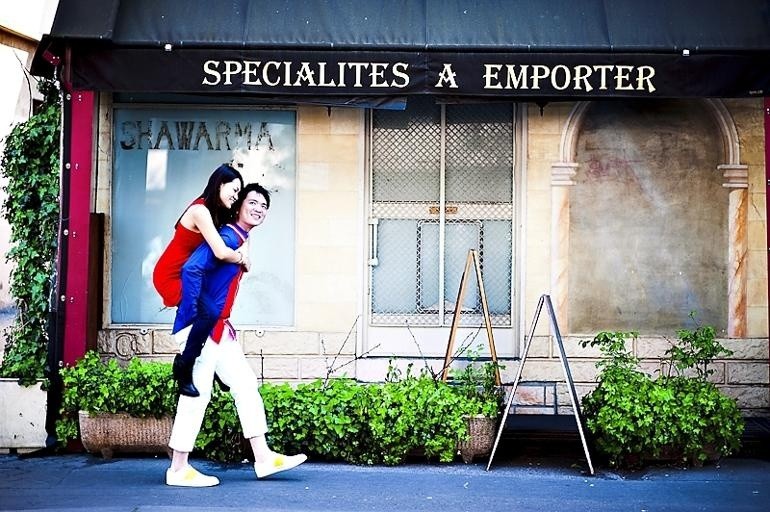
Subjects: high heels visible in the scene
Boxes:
[171,350,200,397]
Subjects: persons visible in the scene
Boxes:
[150,160,253,399]
[163,180,310,490]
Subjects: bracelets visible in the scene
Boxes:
[237,251,247,265]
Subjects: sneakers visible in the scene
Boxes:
[252,451,307,481]
[165,465,220,487]
[212,373,230,393]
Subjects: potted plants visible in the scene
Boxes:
[55,350,506,465]
[577,327,744,469]
